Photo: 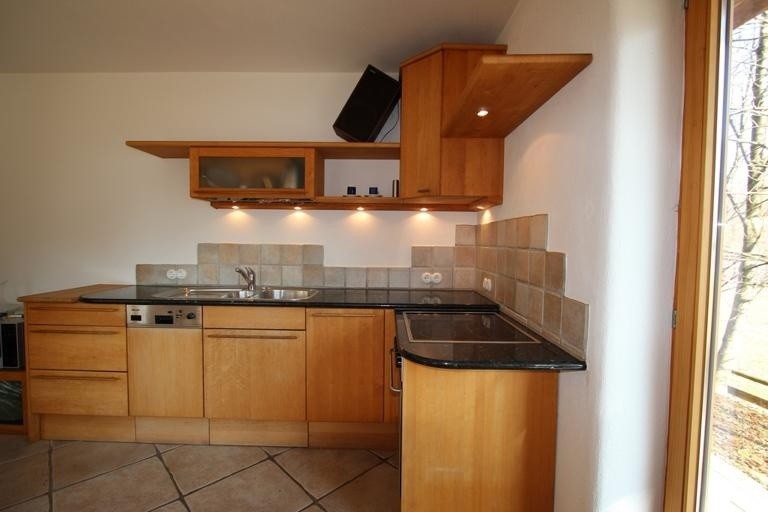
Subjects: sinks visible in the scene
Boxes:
[249,288,320,301]
[221,290,259,299]
[164,292,229,299]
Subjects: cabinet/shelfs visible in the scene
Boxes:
[439,52,591,139]
[203,305,308,448]
[305,308,401,447]
[17,283,133,442]
[126,140,399,210]
[399,44,508,212]
[126,328,206,444]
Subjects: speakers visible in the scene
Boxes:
[332,63,401,143]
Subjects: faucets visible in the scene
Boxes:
[235,266,257,291]
